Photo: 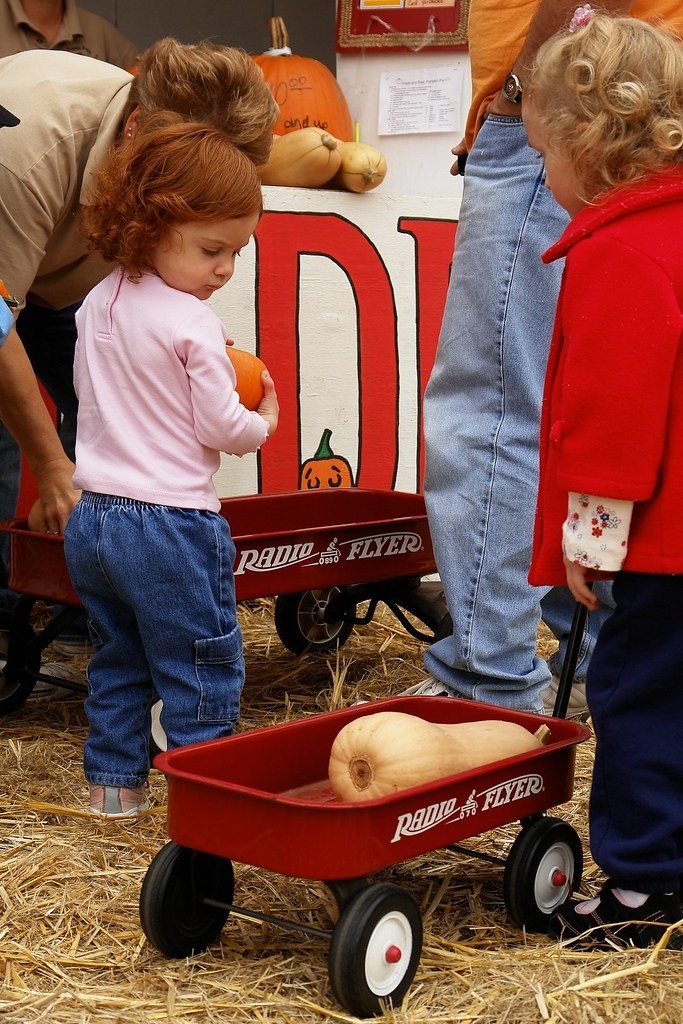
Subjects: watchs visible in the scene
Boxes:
[502,68,523,103]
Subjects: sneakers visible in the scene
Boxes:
[350,676,470,708]
[540,675,588,714]
[90,779,151,826]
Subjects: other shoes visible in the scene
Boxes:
[553,887,663,946]
[51,640,96,659]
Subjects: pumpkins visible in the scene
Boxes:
[329,711,549,802]
[227,345,268,410]
[246,15,388,194]
[27,497,44,533]
[127,66,141,77]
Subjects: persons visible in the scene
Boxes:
[64,112,279,824]
[350,1,681,715]
[523,5,682,946]
[0,0,282,657]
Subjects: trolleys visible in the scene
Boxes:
[138,582,594,1017]
[0,483,457,717]
